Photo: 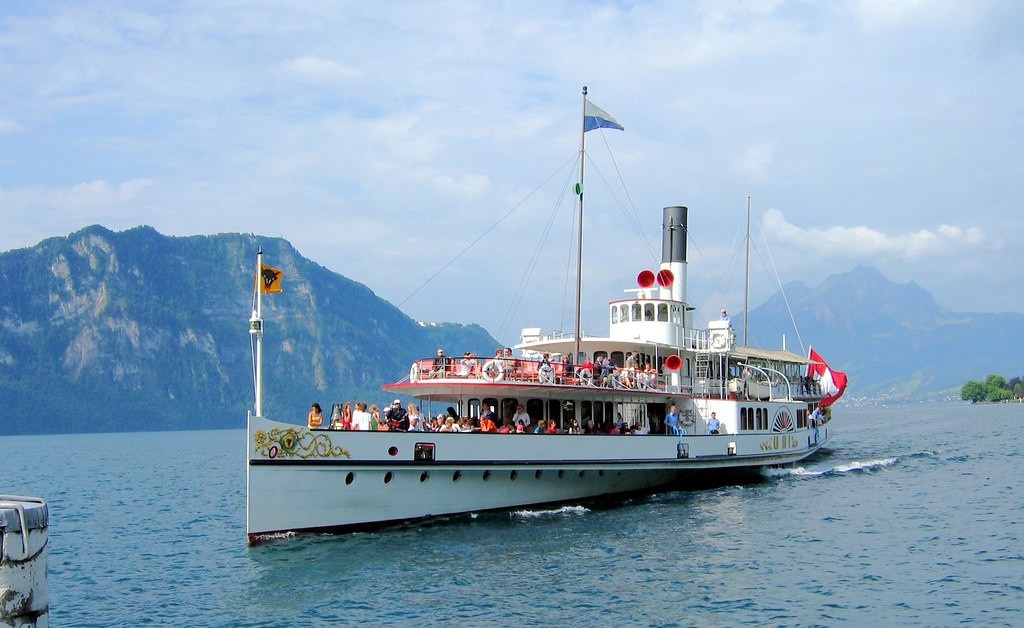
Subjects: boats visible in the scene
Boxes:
[245,86,833,546]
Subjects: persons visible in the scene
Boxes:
[488,348,516,380]
[649,413,663,434]
[742,367,753,401]
[536,353,554,384]
[664,406,682,434]
[562,355,573,377]
[458,352,481,375]
[431,349,448,378]
[728,368,733,379]
[719,310,732,321]
[579,351,658,389]
[706,412,720,434]
[807,405,832,447]
[308,399,647,435]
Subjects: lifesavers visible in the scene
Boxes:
[636,373,646,391]
[538,366,553,385]
[482,360,504,383]
[611,372,622,388]
[580,369,592,387]
[410,363,418,383]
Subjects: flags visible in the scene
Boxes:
[584,99,624,132]
[255,260,283,294]
[806,348,848,409]
